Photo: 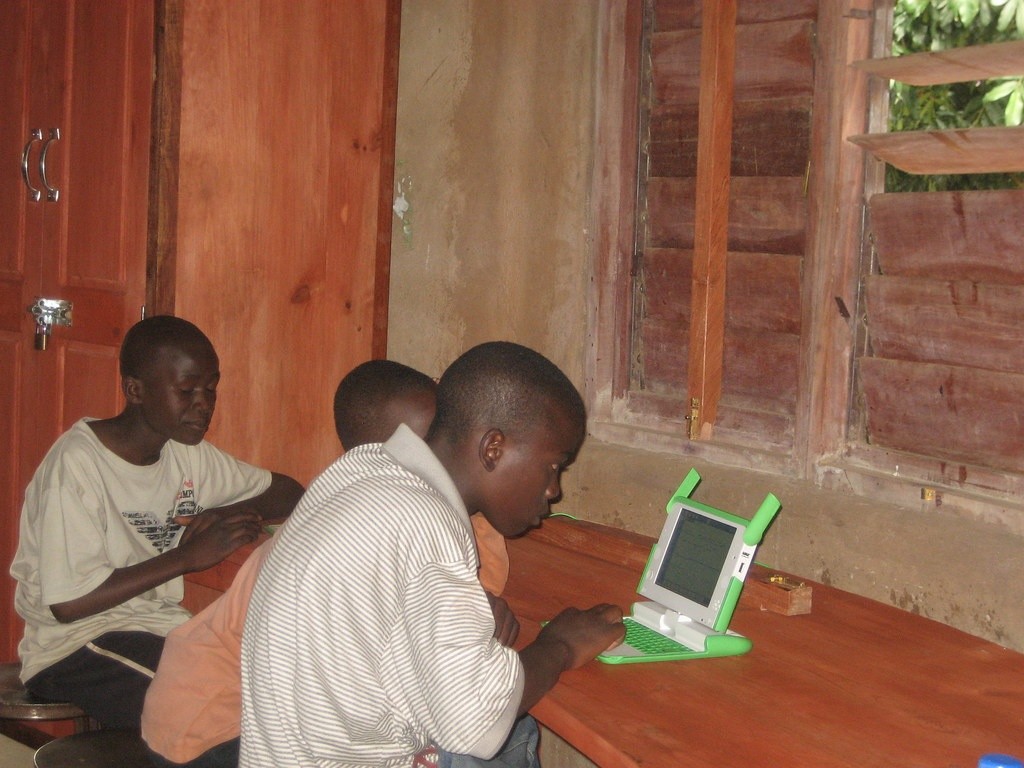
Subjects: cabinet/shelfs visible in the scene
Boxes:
[0,0,156,667]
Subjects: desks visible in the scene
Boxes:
[182,499,1024,768]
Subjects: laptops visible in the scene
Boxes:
[539,466,782,666]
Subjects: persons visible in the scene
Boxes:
[141,360,510,768]
[10,316,306,731]
[237,342,626,767]
